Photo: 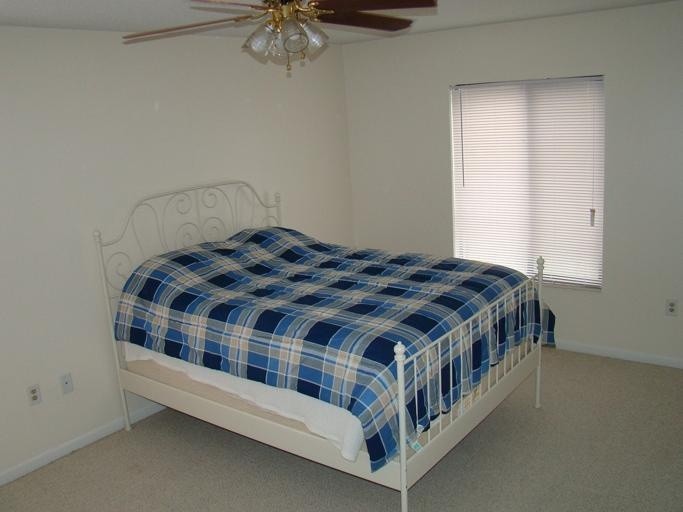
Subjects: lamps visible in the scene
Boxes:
[242,5,331,69]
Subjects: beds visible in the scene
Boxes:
[92,181,556,512]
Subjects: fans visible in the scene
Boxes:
[122,0,438,40]
